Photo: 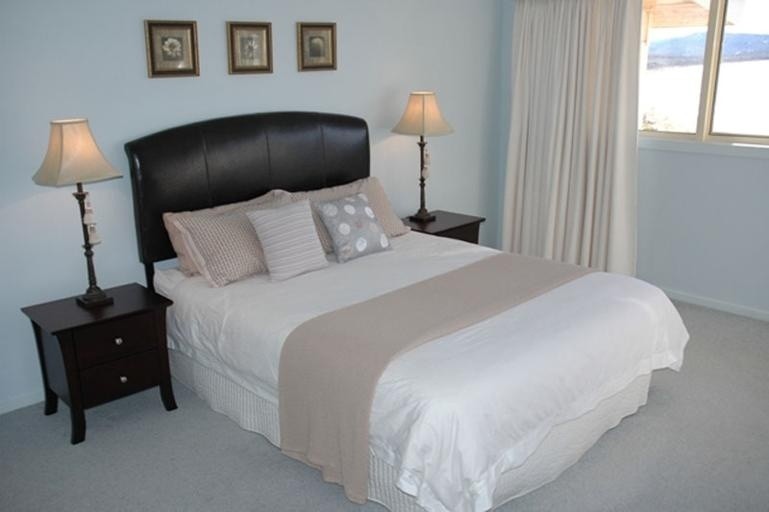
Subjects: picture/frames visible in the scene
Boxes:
[295,20,338,74]
[143,18,202,79]
[225,19,274,77]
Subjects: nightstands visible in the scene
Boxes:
[399,209,487,244]
[20,281,176,446]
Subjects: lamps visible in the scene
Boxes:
[389,91,458,224]
[31,118,127,310]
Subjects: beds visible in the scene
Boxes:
[123,110,691,512]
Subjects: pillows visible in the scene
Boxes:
[163,173,412,290]
